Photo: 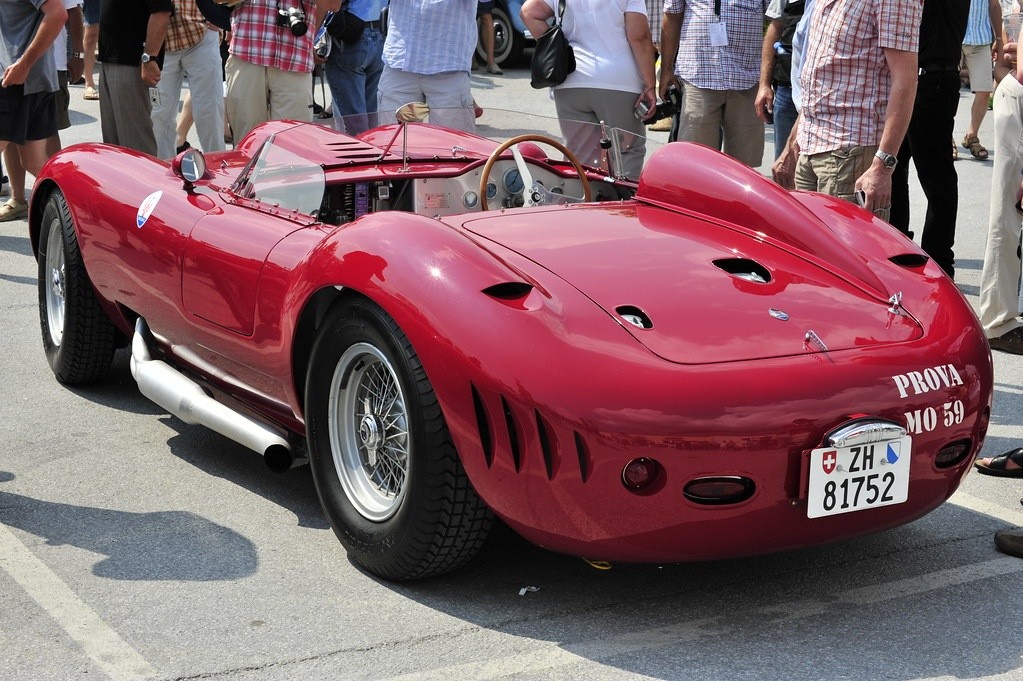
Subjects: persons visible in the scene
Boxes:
[754,0,821,189]
[376,0,480,136]
[226,1,341,162]
[645,0,682,132]
[99,0,175,169]
[0,0,231,200]
[324,0,393,139]
[0,0,69,223]
[794,0,923,223]
[469,0,503,74]
[659,0,771,168]
[891,0,1023,558]
[518,0,657,181]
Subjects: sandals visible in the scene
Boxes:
[962,134,988,161]
[952,139,958,160]
[318,102,333,118]
[974,448,1023,477]
[0,198,28,221]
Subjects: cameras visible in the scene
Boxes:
[634,101,650,120]
[277,7,308,36]
[642,86,683,126]
[856,189,866,207]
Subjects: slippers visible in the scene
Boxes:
[83,85,100,100]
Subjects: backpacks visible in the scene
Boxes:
[771,0,806,87]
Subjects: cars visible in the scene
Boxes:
[473,0,539,68]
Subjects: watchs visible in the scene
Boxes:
[141,53,158,63]
[875,148,898,170]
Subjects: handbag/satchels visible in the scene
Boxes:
[531,0,576,89]
[324,10,365,45]
[195,0,235,31]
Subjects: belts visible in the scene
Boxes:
[363,21,382,28]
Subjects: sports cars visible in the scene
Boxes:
[26,108,993,588]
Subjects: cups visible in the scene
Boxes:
[1002,12,1023,64]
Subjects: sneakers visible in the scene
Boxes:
[487,62,502,74]
[994,527,1023,558]
[472,55,479,69]
[988,326,1023,355]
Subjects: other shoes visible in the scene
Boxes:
[176,141,202,157]
[2,175,8,183]
[69,76,86,85]
[475,108,483,117]
[0,188,9,196]
[313,101,322,114]
[223,131,233,142]
